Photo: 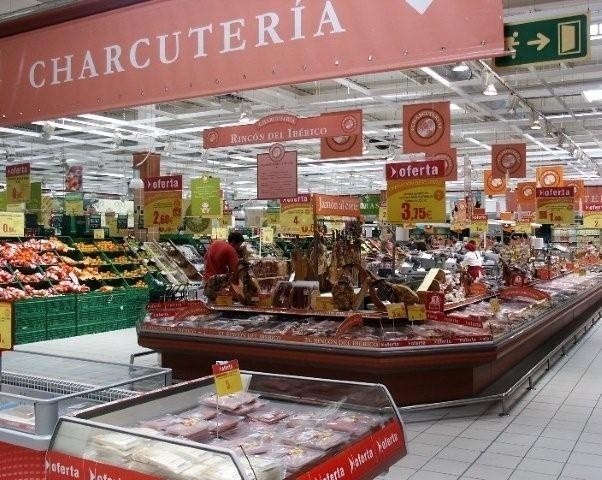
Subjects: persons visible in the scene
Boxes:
[201,231,245,287]
[459,241,485,282]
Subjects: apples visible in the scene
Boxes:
[0,239,81,301]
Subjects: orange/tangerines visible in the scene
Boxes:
[75,240,117,250]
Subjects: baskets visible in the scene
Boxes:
[1,236,168,346]
